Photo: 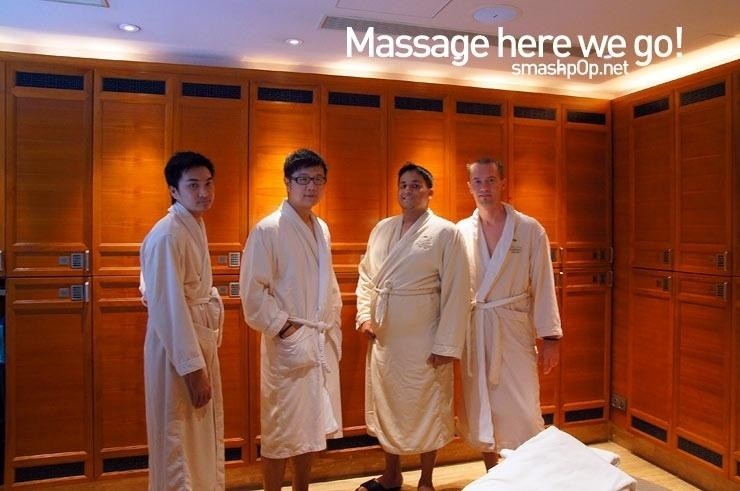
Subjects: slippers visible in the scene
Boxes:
[353,478,400,491]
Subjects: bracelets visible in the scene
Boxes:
[279,323,295,337]
[543,337,560,344]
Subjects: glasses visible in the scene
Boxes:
[290,176,327,186]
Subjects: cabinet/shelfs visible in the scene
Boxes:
[0,52,740,491]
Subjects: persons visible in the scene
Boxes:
[239,146,342,491]
[139,150,228,491]
[353,159,470,491]
[453,159,565,476]
